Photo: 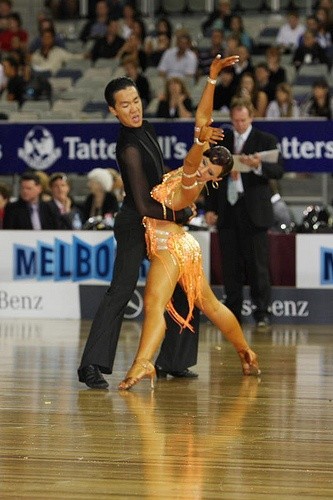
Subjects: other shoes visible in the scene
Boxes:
[253,321,273,335]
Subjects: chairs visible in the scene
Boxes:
[0,0,333,118]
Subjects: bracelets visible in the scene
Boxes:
[208,77,217,85]
[195,138,207,146]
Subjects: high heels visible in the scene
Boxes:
[236,345,259,376]
[77,365,110,389]
[154,364,199,380]
[118,359,156,391]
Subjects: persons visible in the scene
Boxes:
[116,55,262,391]
[0,167,293,230]
[76,76,202,389]
[203,97,285,328]
[0,0,333,118]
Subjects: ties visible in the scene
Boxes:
[227,134,244,207]
[30,204,41,230]
